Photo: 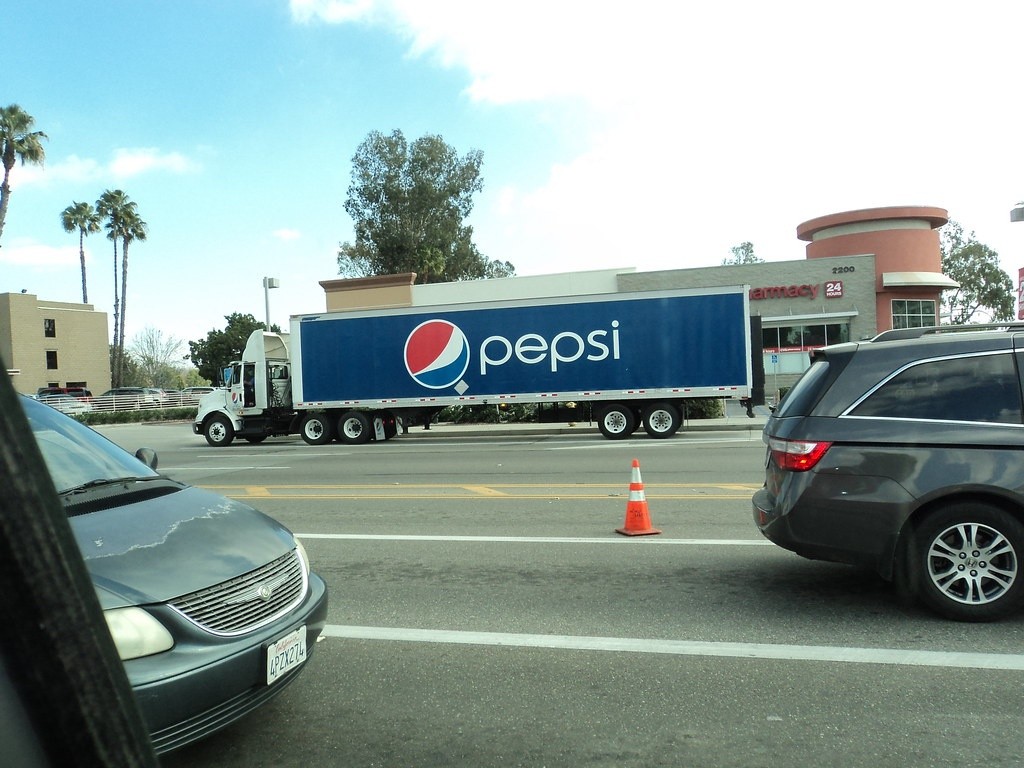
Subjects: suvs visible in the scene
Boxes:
[96,386,219,410]
[752,320,1023,624]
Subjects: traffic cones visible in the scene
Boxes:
[614,459,662,537]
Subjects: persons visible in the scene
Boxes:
[273,365,281,378]
[244,368,254,407]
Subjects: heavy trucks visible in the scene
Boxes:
[191,283,756,448]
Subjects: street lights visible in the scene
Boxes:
[263,276,279,331]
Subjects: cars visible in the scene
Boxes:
[37,387,92,406]
[11,391,329,757]
